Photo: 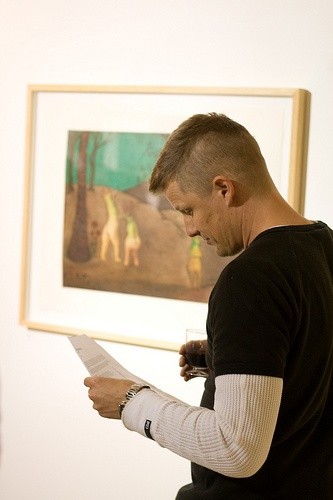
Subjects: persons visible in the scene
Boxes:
[84,113,333,500]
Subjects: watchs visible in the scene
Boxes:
[118,384,150,420]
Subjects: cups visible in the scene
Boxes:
[184,328,208,377]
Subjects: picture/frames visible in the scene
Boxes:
[18,83,306,353]
